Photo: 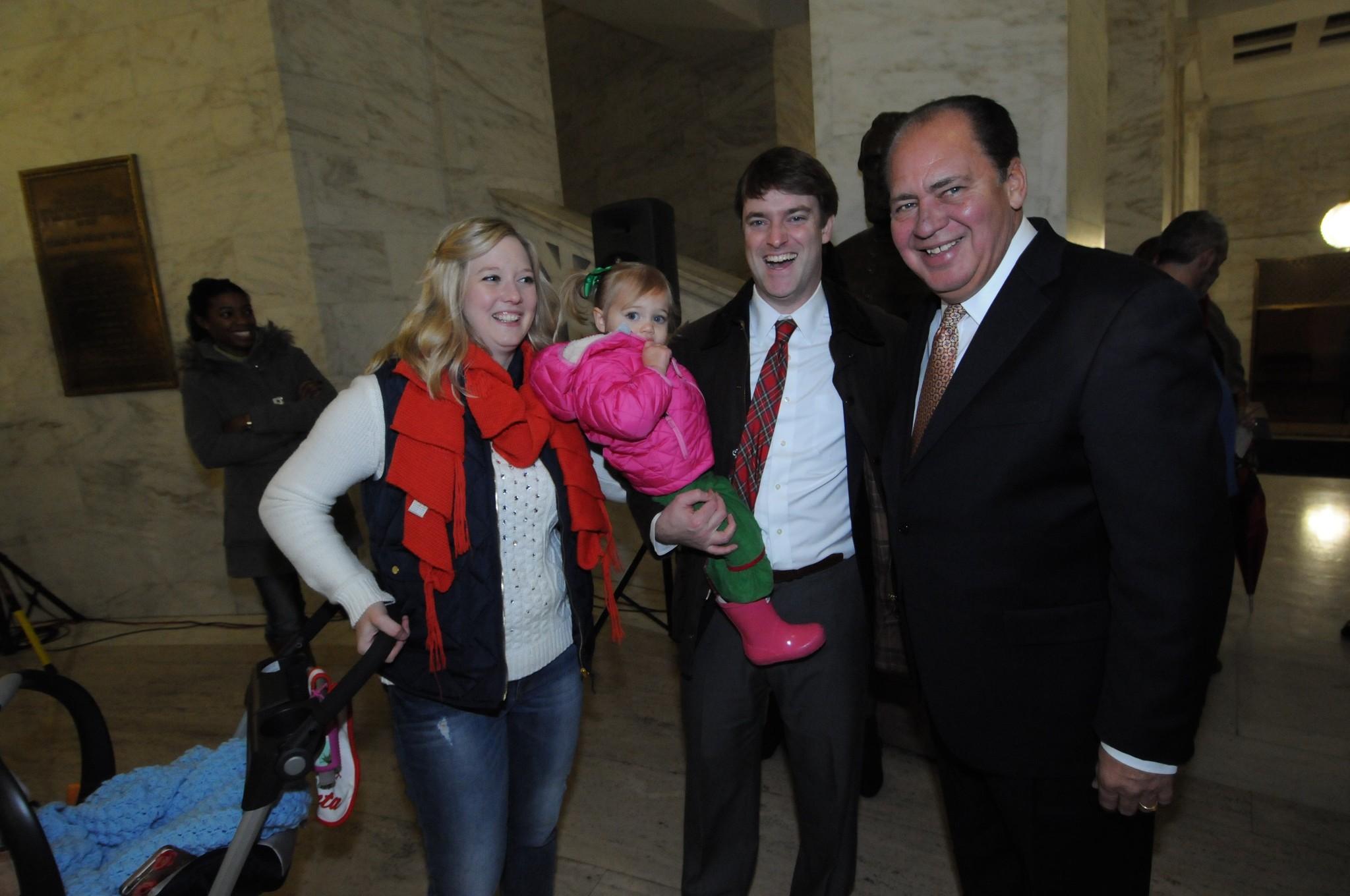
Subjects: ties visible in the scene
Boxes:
[914,304,967,454]
[729,319,796,518]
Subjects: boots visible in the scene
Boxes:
[716,593,825,665]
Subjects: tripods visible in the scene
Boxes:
[1,554,84,672]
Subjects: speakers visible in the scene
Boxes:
[593,198,682,331]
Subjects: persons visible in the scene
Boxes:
[609,96,1263,896]
[180,279,365,688]
[256,216,628,896]
[529,259,825,666]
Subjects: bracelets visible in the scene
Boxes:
[245,414,253,429]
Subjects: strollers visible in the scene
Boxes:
[0,585,406,896]
[575,539,937,799]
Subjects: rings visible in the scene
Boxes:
[1139,801,1158,812]
